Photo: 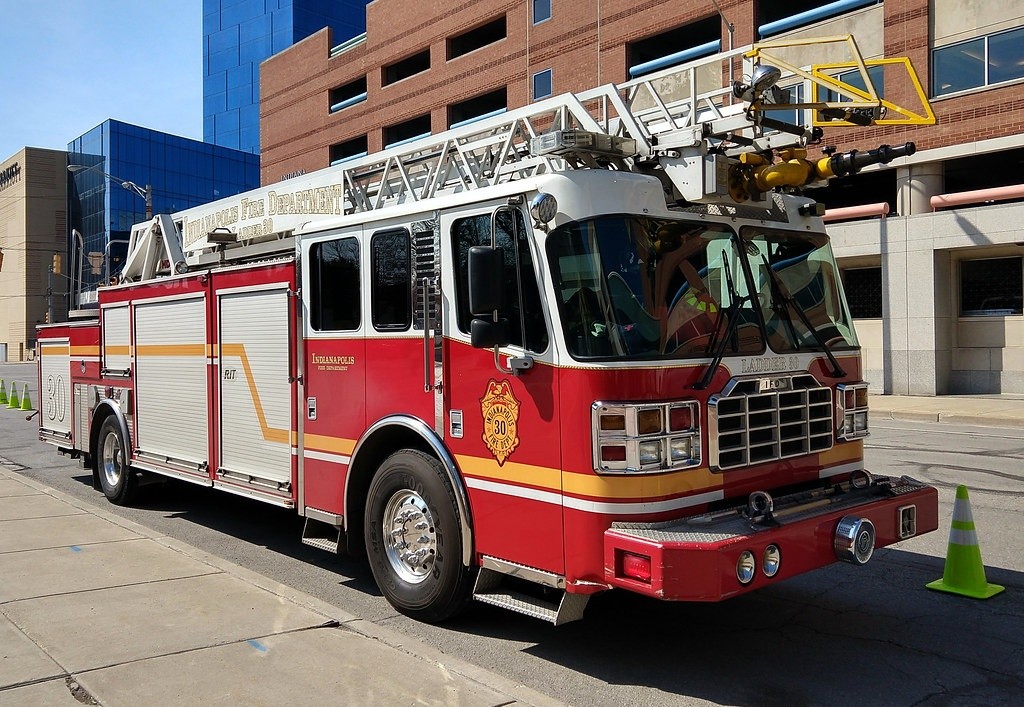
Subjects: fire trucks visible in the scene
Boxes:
[25,34,938,629]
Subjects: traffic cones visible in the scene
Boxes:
[0,379,9,404]
[6,381,21,409]
[925,484,1005,598]
[17,383,36,411]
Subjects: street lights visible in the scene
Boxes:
[66,165,152,220]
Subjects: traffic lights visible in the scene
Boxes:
[110,277,117,286]
[45,312,49,323]
